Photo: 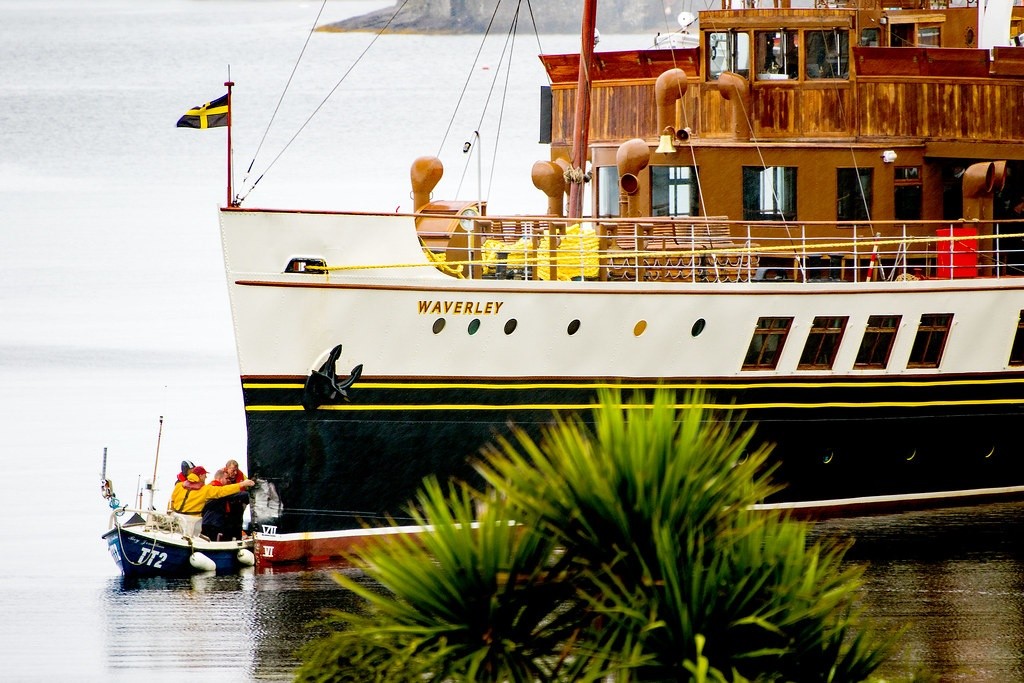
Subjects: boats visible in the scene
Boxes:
[175,0,1024,565]
[99,415,253,578]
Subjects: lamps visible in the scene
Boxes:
[654,125,678,157]
[880,150,897,162]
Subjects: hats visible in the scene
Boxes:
[193,466,210,476]
[181,461,195,475]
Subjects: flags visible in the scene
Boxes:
[177,93,228,130]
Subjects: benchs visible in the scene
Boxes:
[484,216,761,280]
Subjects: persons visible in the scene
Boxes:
[168,460,255,541]
